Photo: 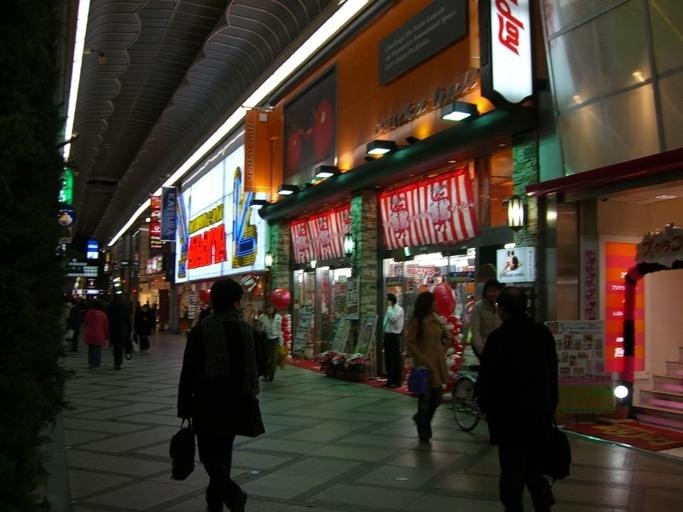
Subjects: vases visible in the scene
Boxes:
[334,368,349,380]
[324,367,336,377]
[348,370,368,382]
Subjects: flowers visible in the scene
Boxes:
[315,349,337,367]
[332,352,354,370]
[345,352,371,373]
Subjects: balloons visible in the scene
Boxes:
[272,288,292,358]
[433,282,464,403]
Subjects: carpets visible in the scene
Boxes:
[567,419,683,451]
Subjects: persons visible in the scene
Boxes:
[510,251,519,270]
[477,286,560,512]
[382,293,405,388]
[406,291,450,446]
[177,278,266,512]
[260,304,283,382]
[67,293,157,370]
[471,279,503,360]
[505,251,512,269]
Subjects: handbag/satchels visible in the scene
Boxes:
[408,368,429,392]
[540,424,572,479]
[170,427,195,479]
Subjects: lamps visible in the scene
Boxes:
[507,194,525,232]
[315,165,338,178]
[366,140,396,155]
[343,232,356,257]
[277,184,297,197]
[441,102,478,123]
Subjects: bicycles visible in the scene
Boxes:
[449,364,485,433]
